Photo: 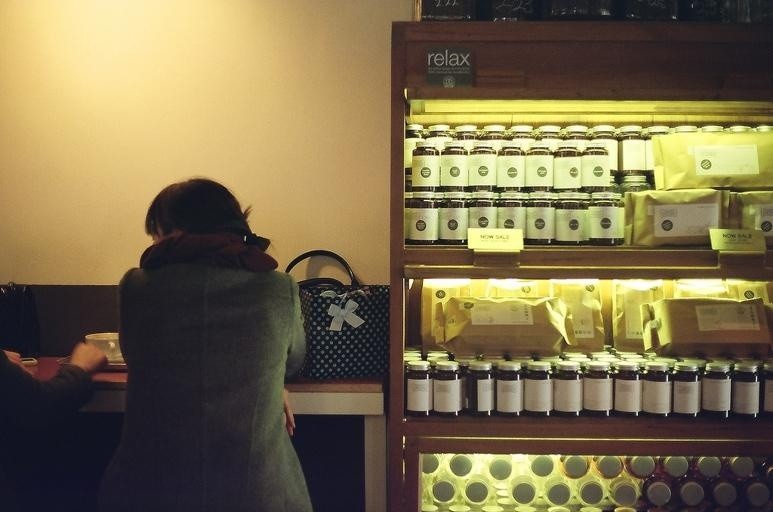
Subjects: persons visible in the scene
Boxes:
[98,175,317,512]
[0,333,111,510]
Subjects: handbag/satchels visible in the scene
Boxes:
[285,249,389,380]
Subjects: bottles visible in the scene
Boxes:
[403,345,773,420]
[403,120,773,247]
[420,453,773,512]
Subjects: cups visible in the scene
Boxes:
[85,332,120,359]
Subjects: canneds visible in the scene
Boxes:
[417,453,773,512]
[403,345,773,422]
[403,125,773,246]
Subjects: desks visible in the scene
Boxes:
[17,355,385,511]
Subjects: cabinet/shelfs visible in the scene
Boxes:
[383,11,771,510]
